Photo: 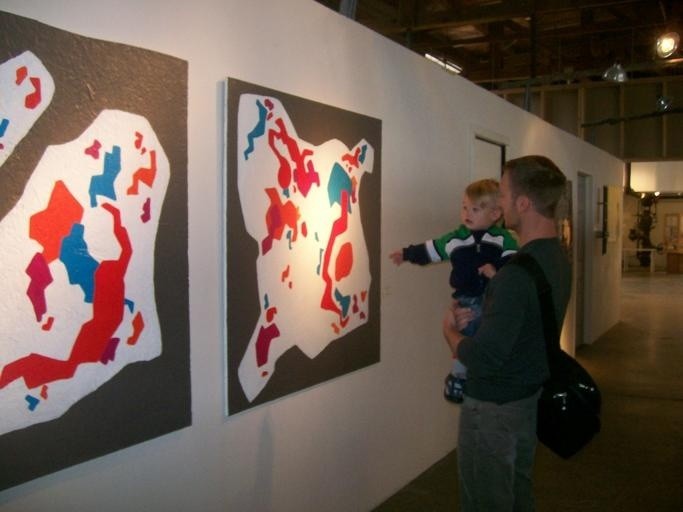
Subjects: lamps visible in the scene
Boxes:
[652,93,675,112]
[652,27,680,59]
[600,59,629,85]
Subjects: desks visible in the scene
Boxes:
[623,248,657,274]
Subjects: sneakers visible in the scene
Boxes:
[444,374,466,404]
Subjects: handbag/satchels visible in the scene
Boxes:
[536,350,601,461]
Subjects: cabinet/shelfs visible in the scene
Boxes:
[666,249,683,274]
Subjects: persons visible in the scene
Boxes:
[443,155,579,511]
[387,179,518,402]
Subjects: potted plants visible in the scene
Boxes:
[627,196,664,269]
